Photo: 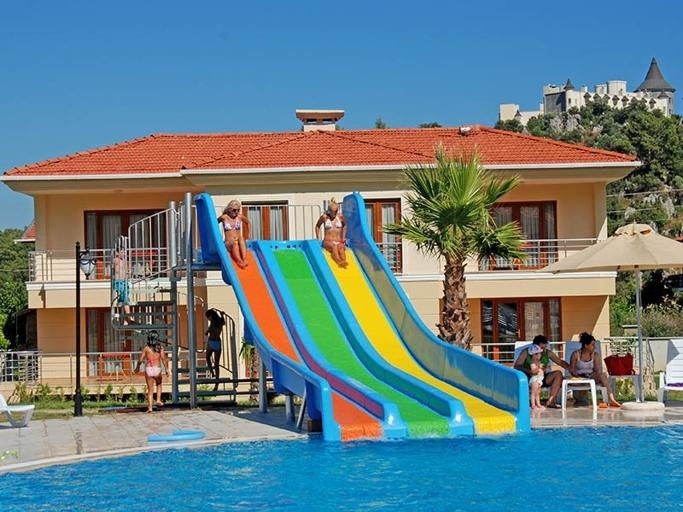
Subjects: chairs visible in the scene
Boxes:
[515,340,558,404]
[564,340,609,405]
[658,339,683,403]
[0,394,35,427]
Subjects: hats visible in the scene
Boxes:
[528,344,544,355]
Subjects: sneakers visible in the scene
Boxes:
[599,399,620,409]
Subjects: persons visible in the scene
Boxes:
[218,200,252,268]
[112,246,134,325]
[527,344,545,410]
[205,310,225,391]
[134,330,169,413]
[514,335,576,409]
[570,332,621,407]
[315,197,348,266]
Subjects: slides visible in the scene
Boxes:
[193,193,530,444]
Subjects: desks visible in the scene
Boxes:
[608,375,641,403]
[561,378,597,411]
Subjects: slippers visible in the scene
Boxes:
[532,403,562,410]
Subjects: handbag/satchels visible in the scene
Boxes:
[604,351,633,376]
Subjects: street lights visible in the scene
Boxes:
[70,240,98,416]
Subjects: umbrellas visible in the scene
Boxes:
[536,220,683,403]
